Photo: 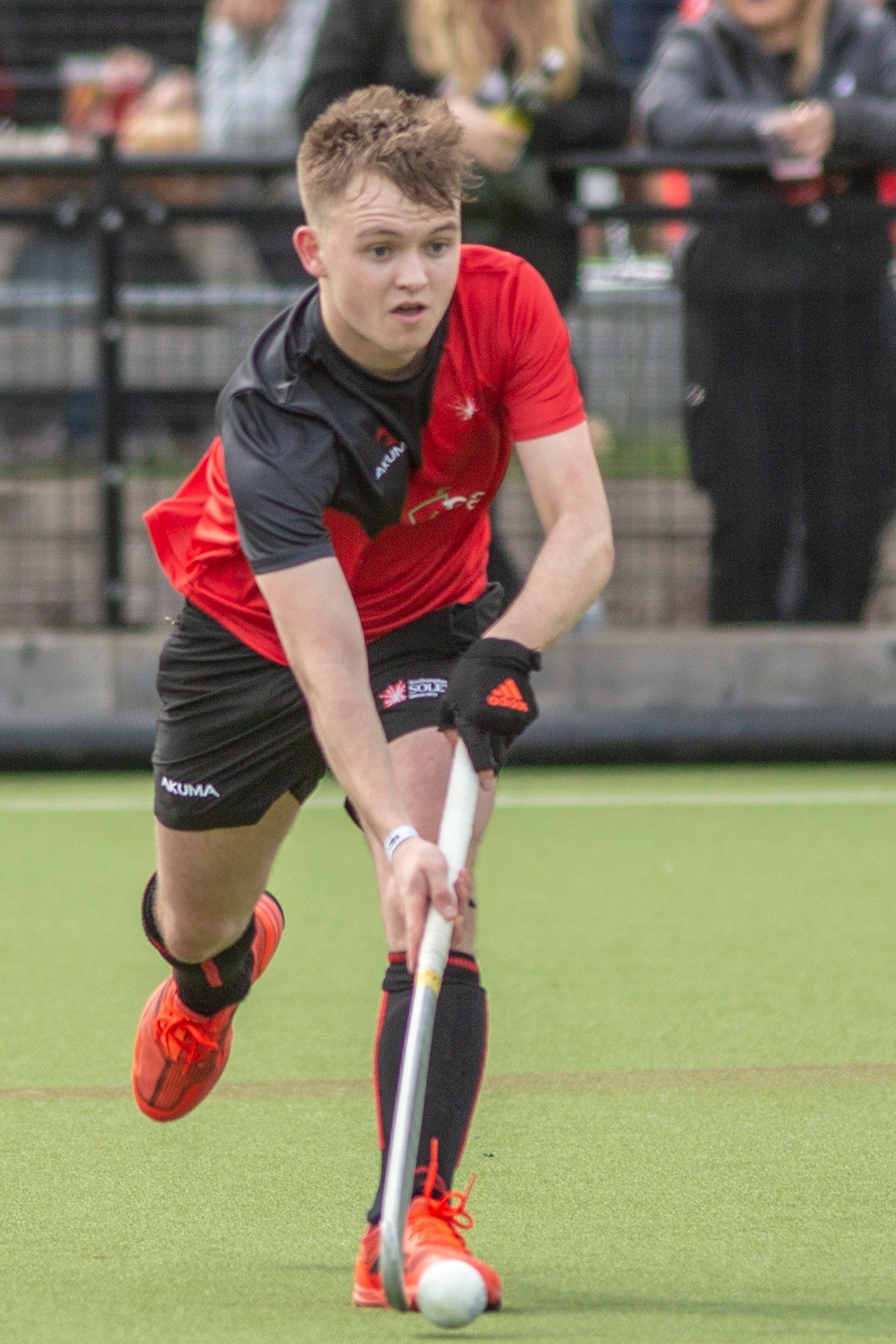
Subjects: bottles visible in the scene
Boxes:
[492,47,567,150]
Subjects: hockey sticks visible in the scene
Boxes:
[376,736,489,1315]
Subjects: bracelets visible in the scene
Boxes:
[384,824,418,863]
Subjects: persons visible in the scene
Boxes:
[0,35,198,282]
[131,89,614,1311]
[630,0,896,627]
[301,0,628,627]
[572,0,681,289]
[171,0,336,288]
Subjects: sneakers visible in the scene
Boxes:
[130,889,286,1122]
[352,1136,505,1314]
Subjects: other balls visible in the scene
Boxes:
[414,1260,488,1329]
